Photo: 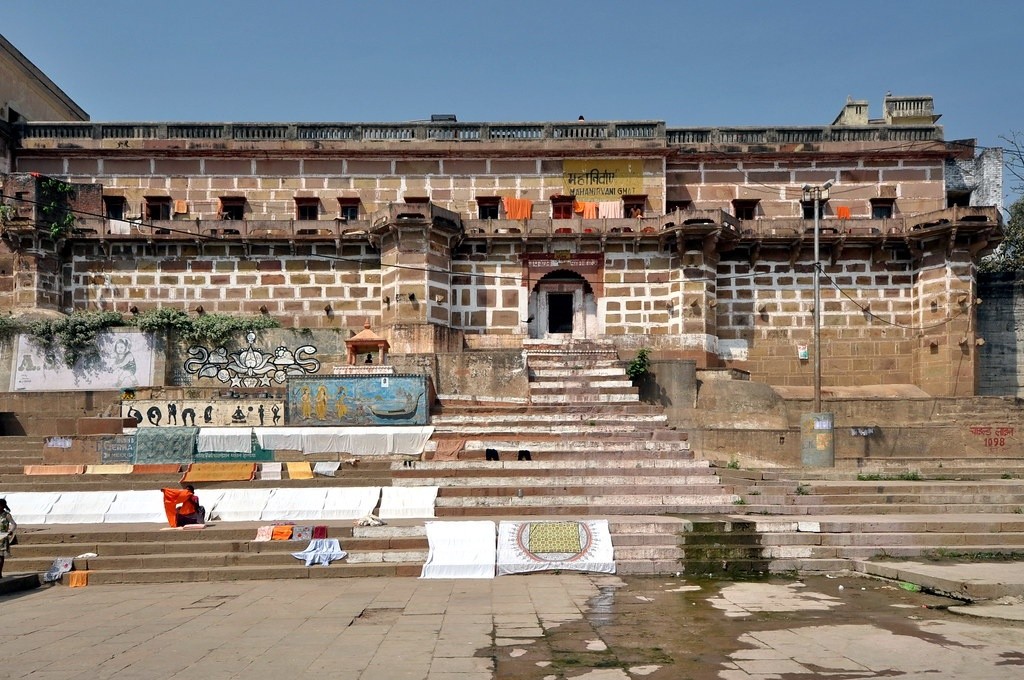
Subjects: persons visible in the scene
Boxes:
[628,205,640,218]
[161,485,200,526]
[0,498,17,578]
